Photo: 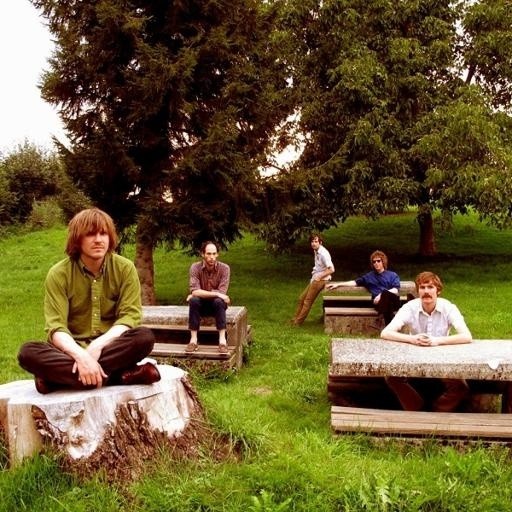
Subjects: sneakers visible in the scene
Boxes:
[219,344,229,354]
[184,343,199,354]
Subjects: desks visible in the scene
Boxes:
[140,305,247,367]
[324,280,415,307]
[330,337,512,414]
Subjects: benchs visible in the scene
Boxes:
[247,324,251,344]
[148,343,236,371]
[331,405,512,446]
[324,307,398,334]
[327,372,505,412]
[323,295,408,308]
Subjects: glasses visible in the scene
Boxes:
[372,260,382,263]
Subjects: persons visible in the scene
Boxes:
[15,206,161,394]
[379,270,472,413]
[186,240,230,353]
[293,232,336,326]
[324,250,408,326]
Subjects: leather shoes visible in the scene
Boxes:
[121,362,162,385]
[35,375,69,394]
